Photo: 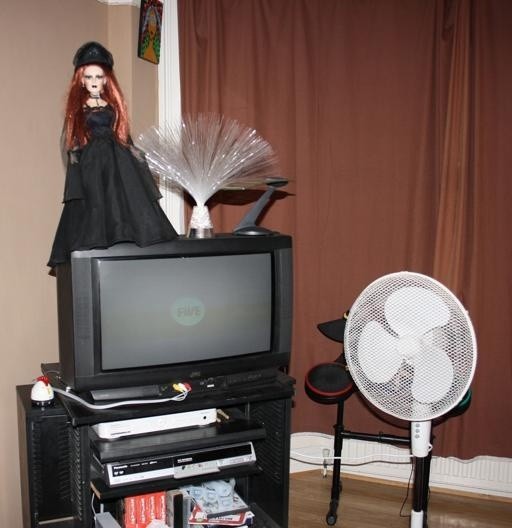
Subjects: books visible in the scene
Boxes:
[118,491,167,528]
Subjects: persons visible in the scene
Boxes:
[47,41,179,277]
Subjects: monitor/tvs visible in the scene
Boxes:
[68,228,295,399]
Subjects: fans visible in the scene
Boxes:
[343,270,479,526]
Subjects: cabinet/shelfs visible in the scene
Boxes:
[15,362,299,527]
